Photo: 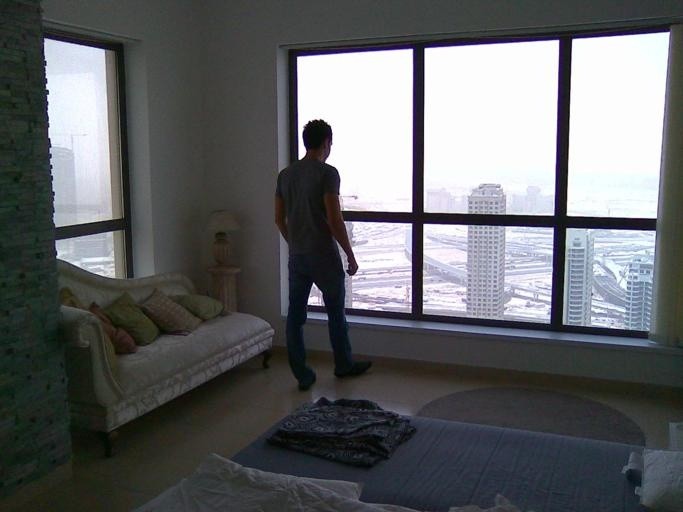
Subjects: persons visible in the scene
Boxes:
[272,118,374,392]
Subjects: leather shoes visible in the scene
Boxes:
[298,373,316,391]
[334,360,373,377]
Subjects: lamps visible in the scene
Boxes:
[201,208,243,265]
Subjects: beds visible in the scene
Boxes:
[131,401,644,511]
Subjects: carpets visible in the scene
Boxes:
[414,386,645,446]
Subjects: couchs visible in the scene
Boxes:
[56,260,275,458]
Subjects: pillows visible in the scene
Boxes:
[639,448,682,510]
[59,283,231,354]
